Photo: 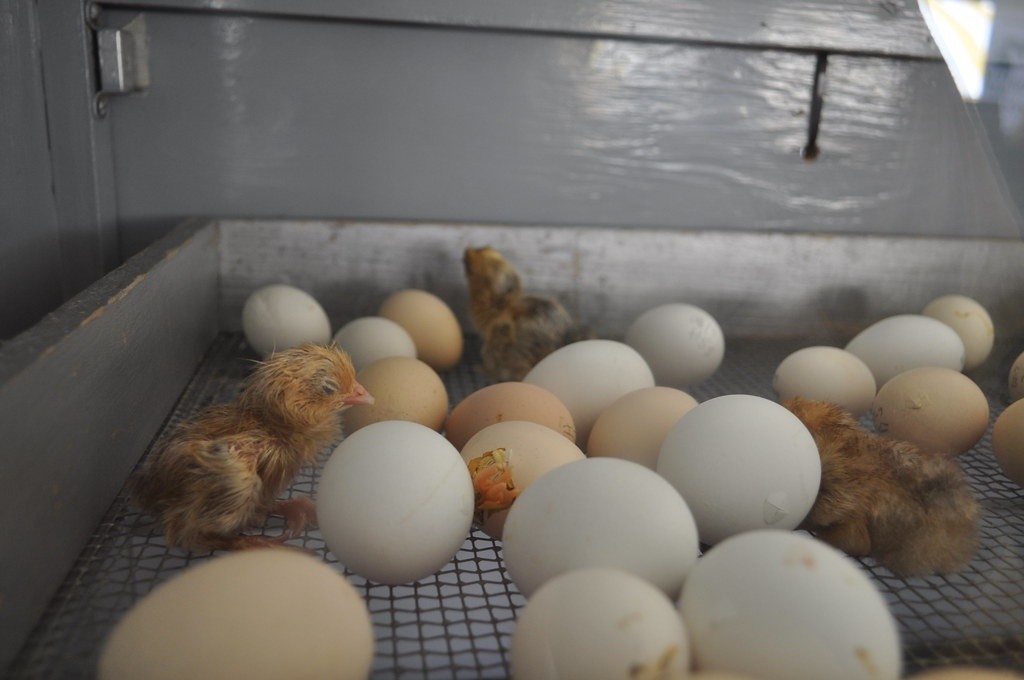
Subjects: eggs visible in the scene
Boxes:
[94,283,1022,680]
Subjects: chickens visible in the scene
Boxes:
[132,345,376,556]
[463,244,583,384]
[783,401,980,580]
